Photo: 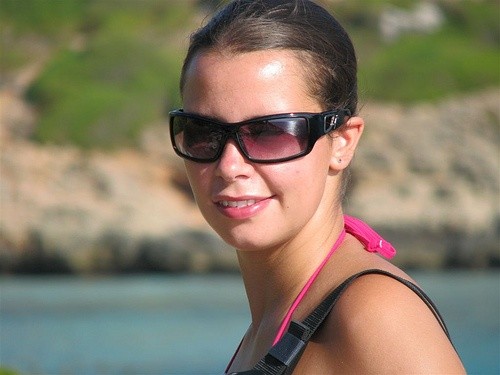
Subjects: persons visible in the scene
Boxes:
[168,0,467,375]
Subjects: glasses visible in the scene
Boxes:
[169,111,347,164]
